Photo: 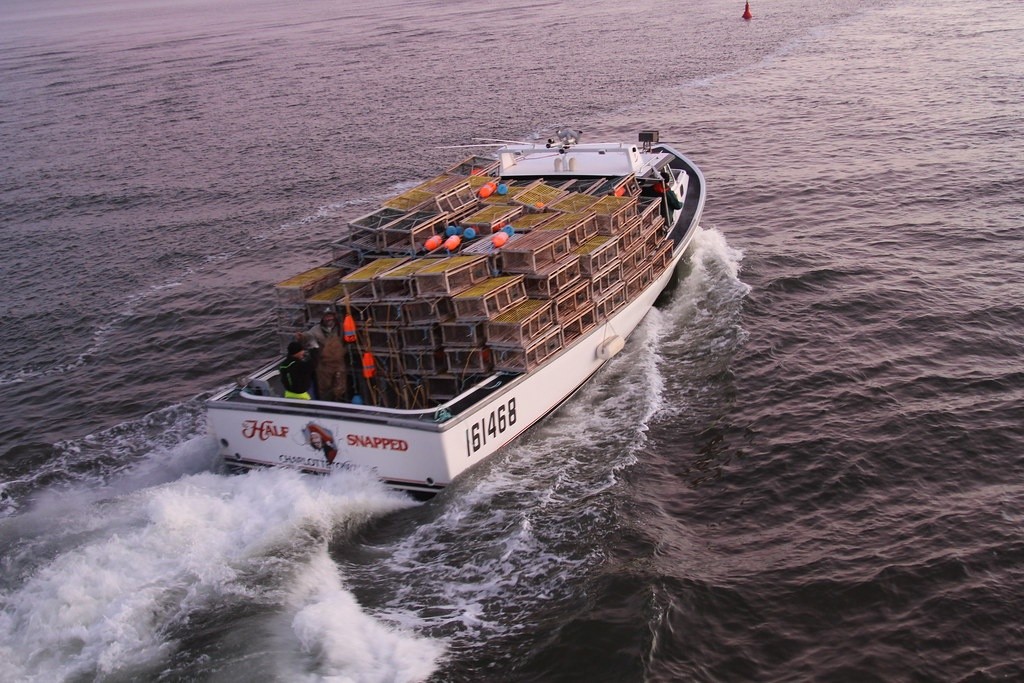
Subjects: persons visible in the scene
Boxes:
[642,173,683,227]
[294,308,347,402]
[279,342,314,400]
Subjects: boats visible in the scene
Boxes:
[203,125,707,503]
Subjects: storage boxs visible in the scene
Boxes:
[274,154,677,406]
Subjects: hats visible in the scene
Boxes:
[288,343,305,355]
[323,306,336,315]
